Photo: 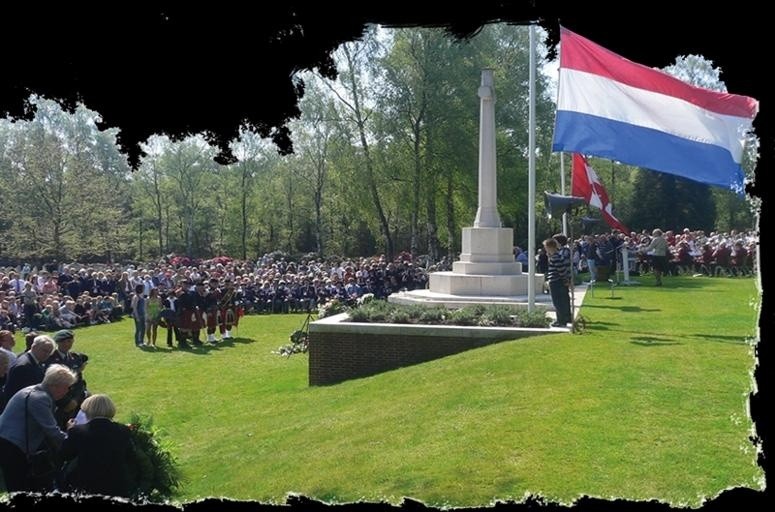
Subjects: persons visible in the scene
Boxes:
[139,249,459,357]
[0,259,139,498]
[514,226,757,286]
[551,233,573,323]
[542,238,570,328]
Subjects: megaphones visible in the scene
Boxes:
[542,188,585,222]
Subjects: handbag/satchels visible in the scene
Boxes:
[27,450,51,480]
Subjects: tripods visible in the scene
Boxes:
[286,312,316,360]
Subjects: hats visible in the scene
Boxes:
[52,330,75,342]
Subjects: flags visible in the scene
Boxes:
[551,23,758,196]
[567,149,633,238]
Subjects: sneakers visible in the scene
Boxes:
[178,336,233,350]
[552,321,566,328]
